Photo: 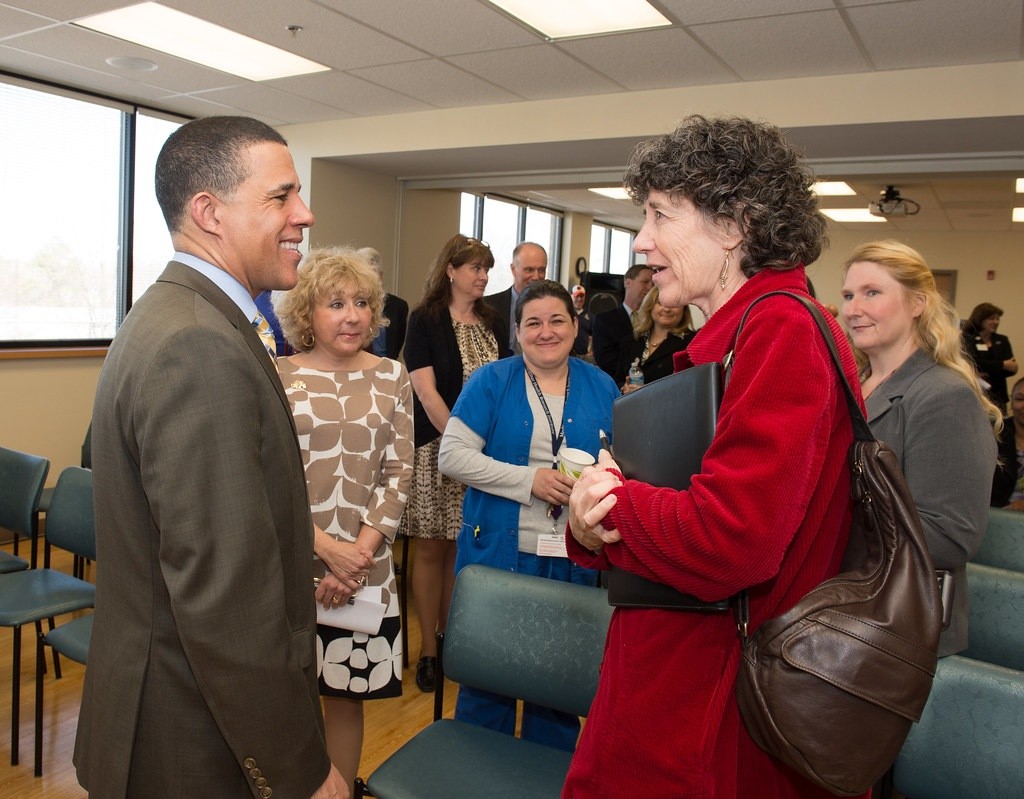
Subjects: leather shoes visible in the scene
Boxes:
[436,635,445,659]
[417,651,438,691]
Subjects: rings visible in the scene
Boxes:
[332,597,340,603]
[581,521,589,529]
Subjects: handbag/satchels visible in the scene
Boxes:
[723,293,941,798]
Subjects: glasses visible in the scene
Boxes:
[452,238,490,261]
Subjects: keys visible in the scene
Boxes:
[546,503,554,517]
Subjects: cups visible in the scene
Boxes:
[559,448,596,482]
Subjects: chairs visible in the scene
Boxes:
[883,503,1024,799]
[34,613,100,777]
[0,465,99,766]
[354,563,618,799]
[0,447,51,574]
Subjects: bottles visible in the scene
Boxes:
[628,363,645,387]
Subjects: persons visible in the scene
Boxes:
[437,278,624,755]
[565,265,700,397]
[565,113,869,799]
[959,302,1024,513]
[271,238,415,799]
[71,114,350,799]
[473,242,547,361]
[403,233,514,694]
[357,246,409,360]
[841,237,1005,660]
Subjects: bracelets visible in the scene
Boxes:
[650,335,667,347]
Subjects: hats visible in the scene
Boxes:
[570,284,585,295]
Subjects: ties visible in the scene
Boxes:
[253,310,281,376]
[632,308,642,340]
